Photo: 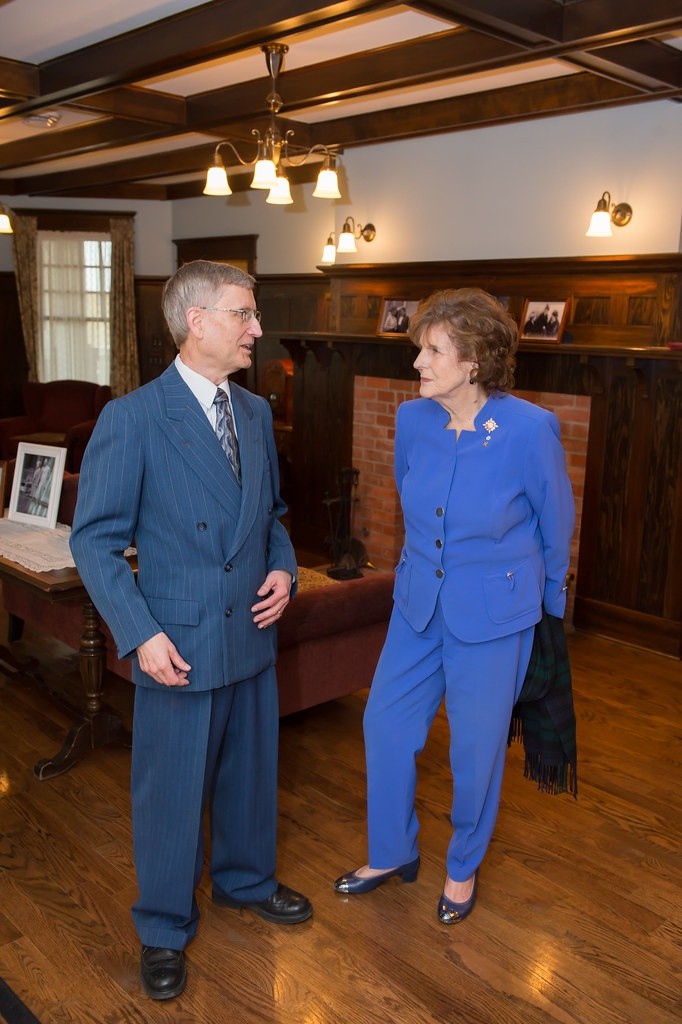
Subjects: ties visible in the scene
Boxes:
[213,387,240,478]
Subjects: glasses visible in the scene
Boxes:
[197,306,263,325]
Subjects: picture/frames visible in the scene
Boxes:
[516,297,572,344]
[7,441,67,530]
[376,297,424,337]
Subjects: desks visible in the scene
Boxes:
[0,507,140,780]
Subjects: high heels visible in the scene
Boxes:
[333,857,422,894]
[438,870,482,925]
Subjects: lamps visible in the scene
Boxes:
[22,109,63,128]
[322,217,376,264]
[584,192,632,238]
[202,42,342,204]
[0,207,14,234]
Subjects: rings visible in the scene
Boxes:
[278,611,282,615]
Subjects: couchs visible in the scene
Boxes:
[2,472,395,719]
[0,380,112,475]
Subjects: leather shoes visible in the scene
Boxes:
[141,946,188,1002]
[212,880,316,924]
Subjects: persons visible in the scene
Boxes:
[72,261,316,1000]
[524,303,560,337]
[383,306,411,334]
[334,286,575,923]
[28,459,51,515]
